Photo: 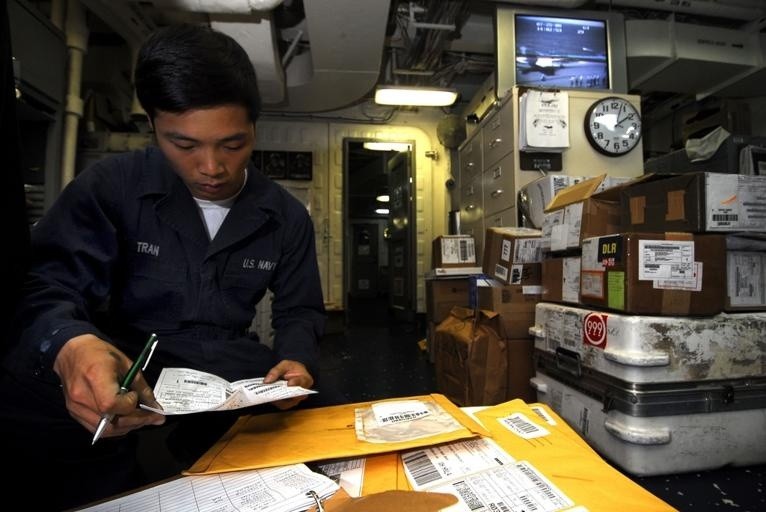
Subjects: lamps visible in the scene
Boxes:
[374,86,458,108]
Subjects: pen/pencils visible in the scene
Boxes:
[91,331,159,446]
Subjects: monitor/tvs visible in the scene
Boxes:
[495,4,629,100]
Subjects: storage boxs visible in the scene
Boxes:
[422,226,543,401]
[544,173,766,313]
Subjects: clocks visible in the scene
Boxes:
[585,96,645,157]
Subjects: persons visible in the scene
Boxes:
[569,73,602,87]
[31,21,329,506]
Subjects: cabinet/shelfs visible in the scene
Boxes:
[456,92,517,258]
